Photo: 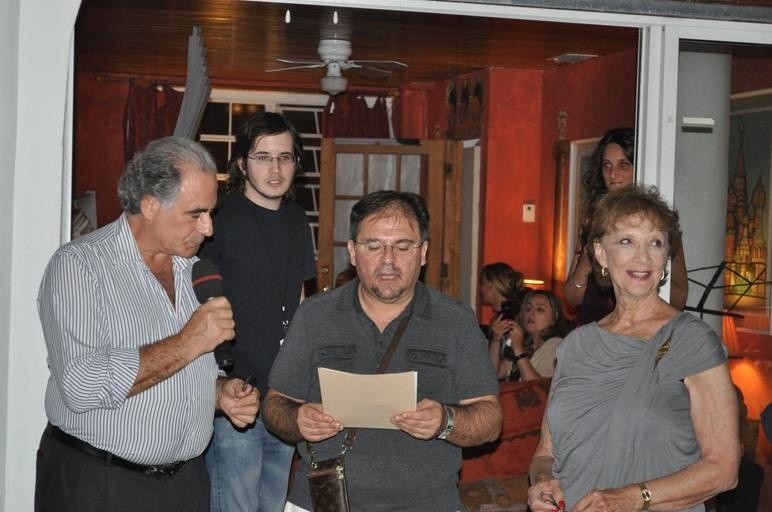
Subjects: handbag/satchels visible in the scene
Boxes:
[303,456,353,511]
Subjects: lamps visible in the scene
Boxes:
[319,62,349,115]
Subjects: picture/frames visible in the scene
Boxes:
[564,136,604,279]
[724,87,771,338]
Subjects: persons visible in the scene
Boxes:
[34,136,262,512]
[527,183,742,512]
[196,112,318,512]
[564,127,634,327]
[262,189,504,512]
[477,263,528,340]
[489,289,572,382]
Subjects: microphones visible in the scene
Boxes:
[190,255,239,371]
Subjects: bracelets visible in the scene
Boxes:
[514,352,529,361]
[639,483,651,511]
[571,275,587,288]
[435,407,454,440]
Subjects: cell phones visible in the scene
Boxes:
[500,301,515,320]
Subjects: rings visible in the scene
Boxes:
[512,330,517,332]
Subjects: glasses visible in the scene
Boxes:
[354,238,422,254]
[245,153,297,165]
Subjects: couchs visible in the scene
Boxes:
[454,377,552,511]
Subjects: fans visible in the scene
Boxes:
[263,39,410,81]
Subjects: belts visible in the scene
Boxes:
[45,420,188,478]
